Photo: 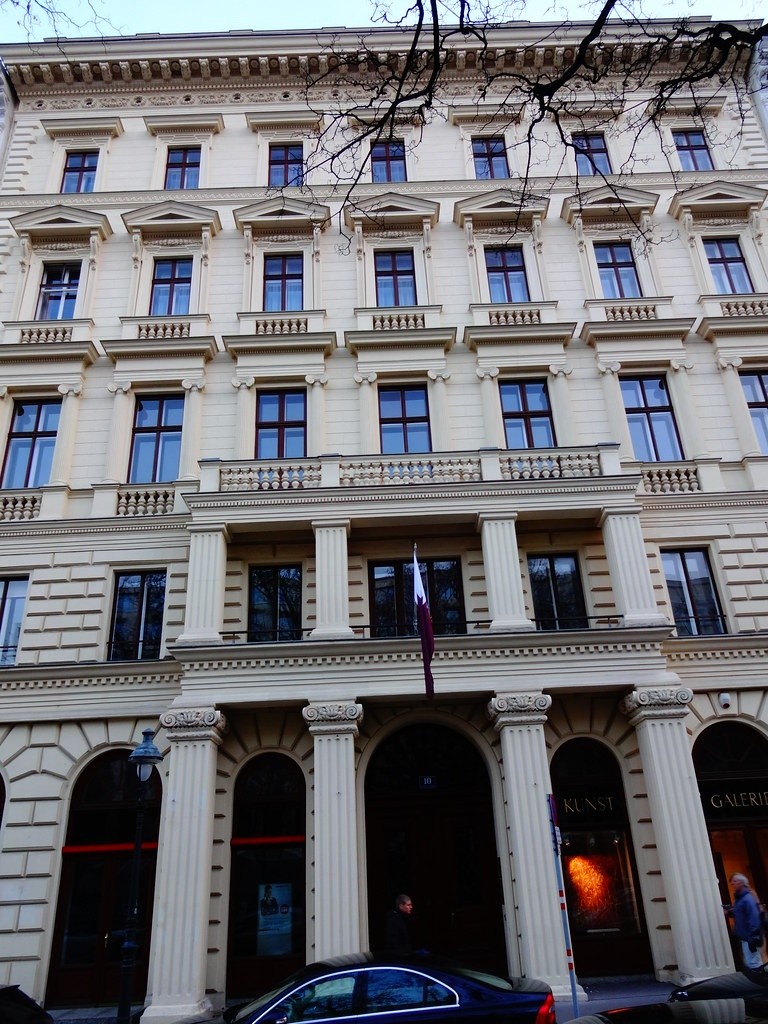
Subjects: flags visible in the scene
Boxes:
[414,556,435,702]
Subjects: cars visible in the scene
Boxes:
[0,985,56,1023]
[559,961,768,1024]
[167,950,556,1024]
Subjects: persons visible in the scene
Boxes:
[261,885,279,914]
[723,873,768,970]
[384,894,417,950]
